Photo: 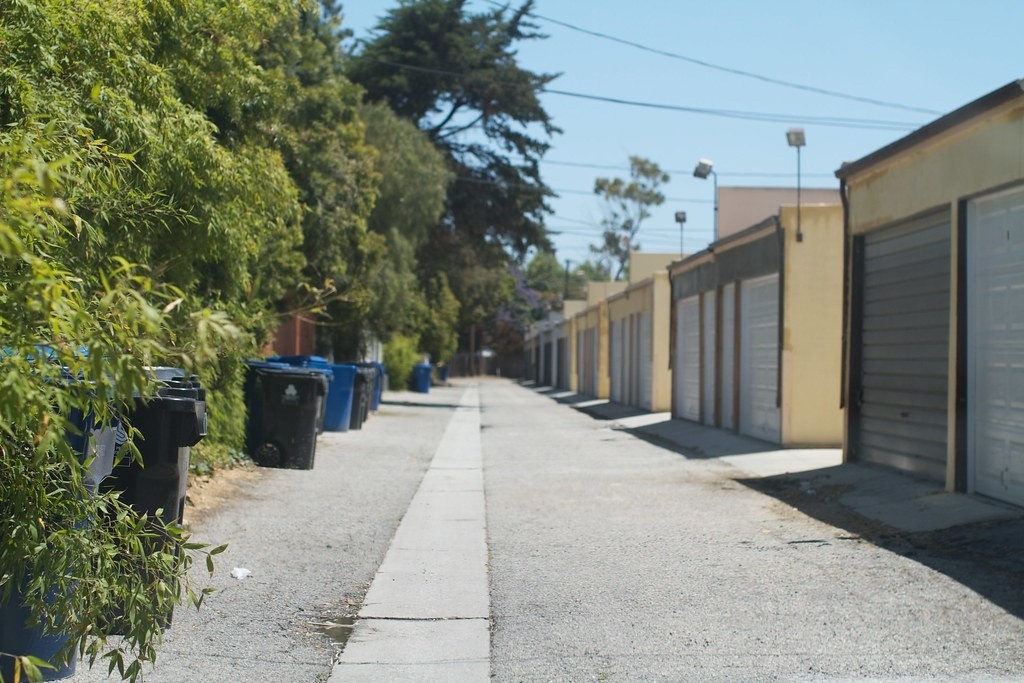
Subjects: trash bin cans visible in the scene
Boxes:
[243,359,291,456]
[87,365,208,635]
[254,368,328,470]
[1,342,135,683]
[265,355,384,432]
[412,365,432,394]
